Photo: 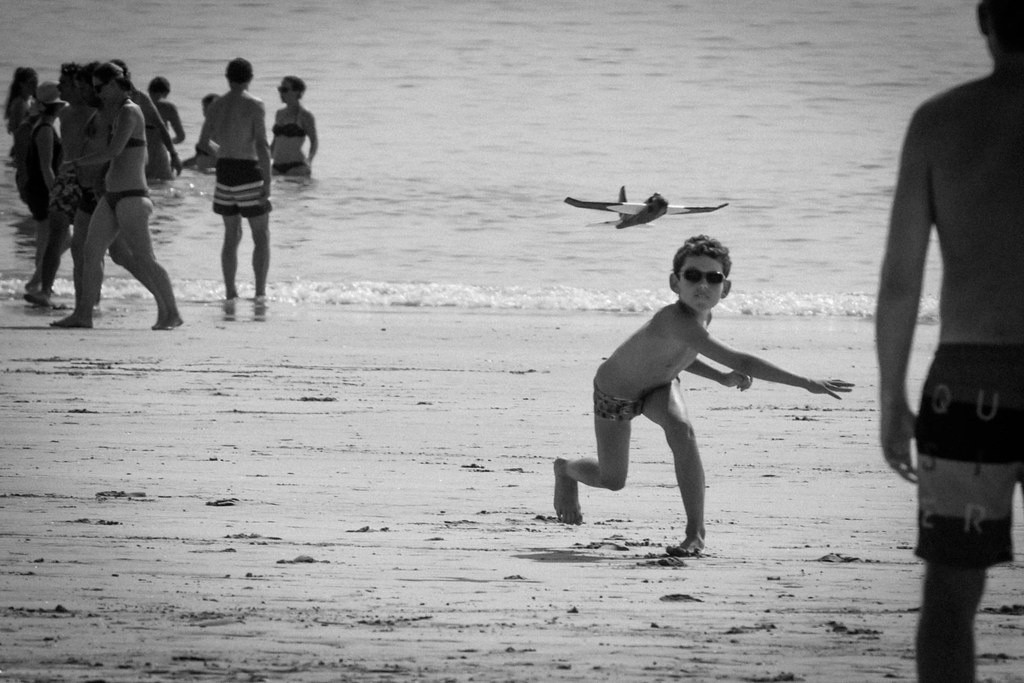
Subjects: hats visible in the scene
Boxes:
[28,82,70,112]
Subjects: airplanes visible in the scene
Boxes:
[562,185,730,230]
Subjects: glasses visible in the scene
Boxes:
[278,87,297,93]
[675,268,725,285]
[92,82,110,94]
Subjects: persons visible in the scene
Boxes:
[876,0,1024,683]
[5,60,185,330]
[196,58,272,299]
[184,94,219,170]
[270,76,318,176]
[554,236,855,556]
[145,77,185,179]
